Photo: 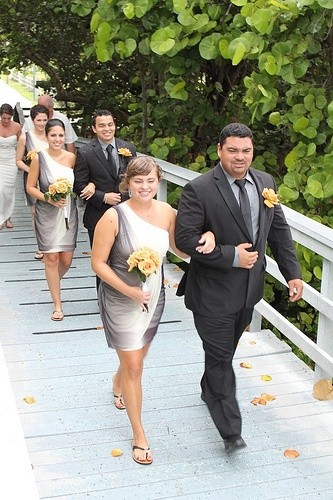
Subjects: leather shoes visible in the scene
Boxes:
[224,435,247,451]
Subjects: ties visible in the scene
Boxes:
[106,144,117,175]
[234,179,253,241]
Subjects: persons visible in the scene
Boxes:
[20,94,78,155]
[0,103,22,229]
[26,119,95,321]
[173,122,304,454]
[16,103,49,259]
[91,156,215,465]
[73,110,138,288]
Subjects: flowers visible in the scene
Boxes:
[117,147,133,158]
[45,177,78,229]
[23,151,37,163]
[126,248,162,311]
[261,187,280,209]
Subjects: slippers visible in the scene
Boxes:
[114,394,126,409]
[51,310,64,321]
[132,445,153,464]
